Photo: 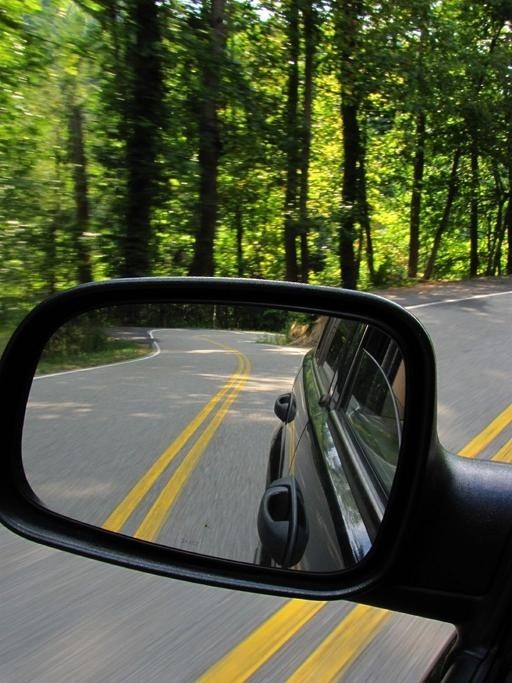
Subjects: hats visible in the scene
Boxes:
[258,317,405,573]
